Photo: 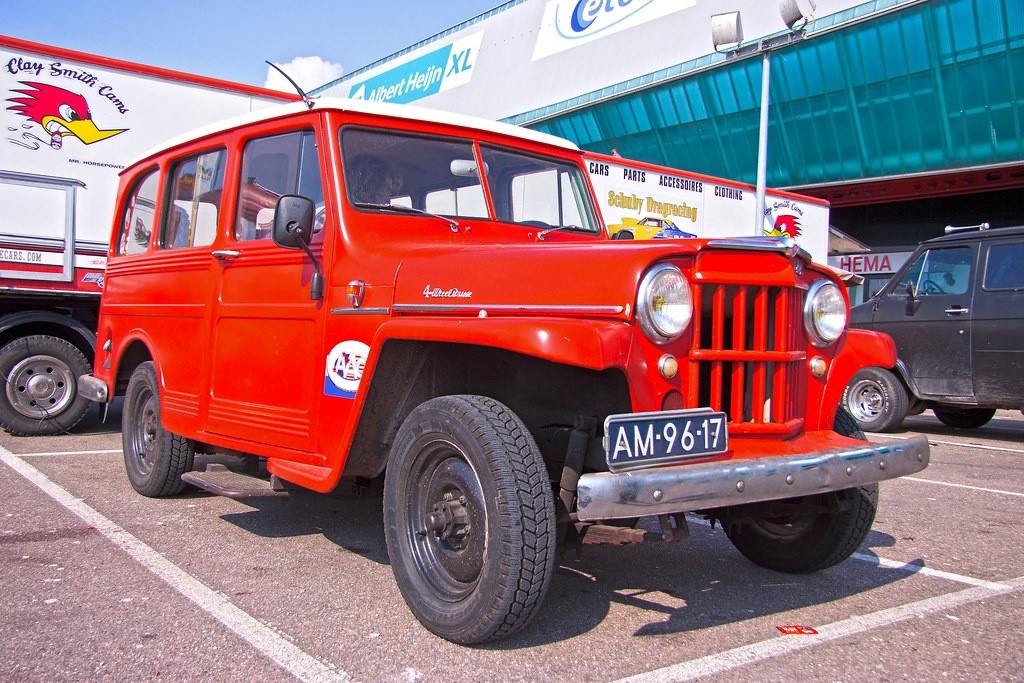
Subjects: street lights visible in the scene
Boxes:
[709,0,816,237]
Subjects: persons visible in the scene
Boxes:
[314,153,403,235]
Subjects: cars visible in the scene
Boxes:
[607,216,697,241]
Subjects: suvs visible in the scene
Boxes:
[849,221,1024,433]
[92,58,932,647]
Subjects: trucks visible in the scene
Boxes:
[0,34,829,436]
